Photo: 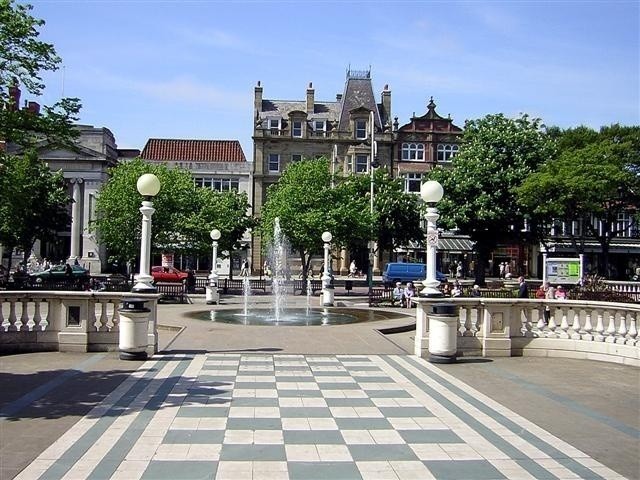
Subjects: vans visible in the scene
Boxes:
[382,263,448,289]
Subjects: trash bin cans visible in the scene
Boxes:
[205,281,218,305]
[323,284,335,306]
[117,298,151,361]
[426,303,461,364]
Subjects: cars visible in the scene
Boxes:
[28,263,90,285]
[151,266,196,285]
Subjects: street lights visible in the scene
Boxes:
[321,231,334,290]
[208,228,221,287]
[131,173,160,293]
[420,180,445,297]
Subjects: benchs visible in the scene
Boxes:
[149,283,184,305]
[186,278,267,294]
[368,288,417,308]
[293,279,334,296]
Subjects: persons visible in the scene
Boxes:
[449,259,474,278]
[626,266,640,282]
[536,284,570,300]
[320,261,325,279]
[347,260,358,278]
[393,282,414,308]
[440,283,481,298]
[183,266,196,293]
[1,258,107,291]
[511,276,530,308]
[498,262,511,279]
[299,261,314,279]
[239,260,248,277]
[262,260,292,280]
